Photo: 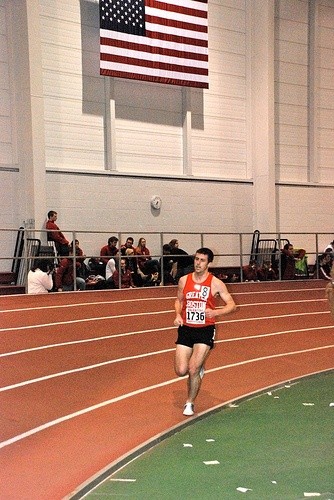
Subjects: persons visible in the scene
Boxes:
[46,211,70,258]
[174,247,238,417]
[53,234,334,291]
[28,259,55,293]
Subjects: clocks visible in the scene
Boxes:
[151,196,162,209]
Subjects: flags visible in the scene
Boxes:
[98,0,210,91]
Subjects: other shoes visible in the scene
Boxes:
[199,366,205,380]
[183,403,194,415]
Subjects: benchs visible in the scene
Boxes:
[83,255,152,284]
